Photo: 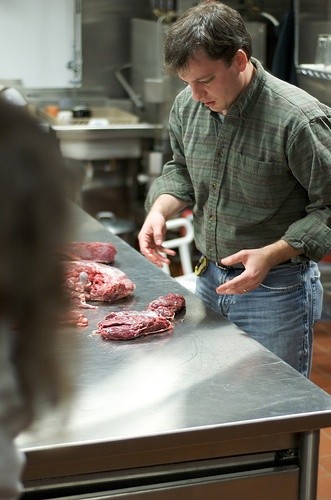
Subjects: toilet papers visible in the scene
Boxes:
[148,152,163,174]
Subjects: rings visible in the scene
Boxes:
[243,289,247,293]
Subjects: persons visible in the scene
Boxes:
[138,1,331,379]
[0,97,74,500]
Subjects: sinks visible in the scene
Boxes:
[49,122,164,159]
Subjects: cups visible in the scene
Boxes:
[314,33,331,71]
